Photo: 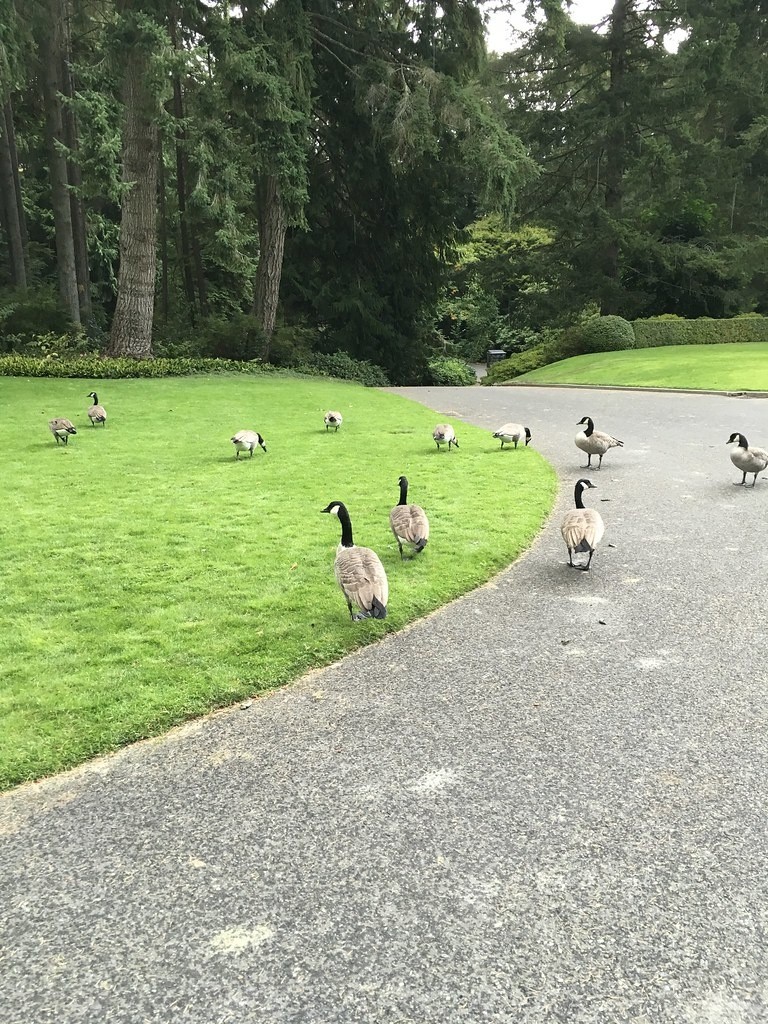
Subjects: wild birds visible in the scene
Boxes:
[49,417,77,446]
[561,478,606,570]
[389,476,430,561]
[86,392,107,427]
[324,410,343,433]
[492,422,532,449]
[726,433,768,488]
[319,501,389,622]
[432,423,459,453]
[231,429,266,460]
[574,417,623,470]
